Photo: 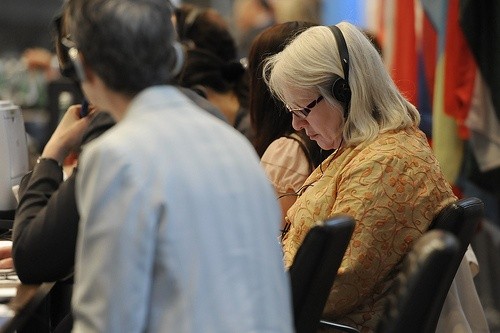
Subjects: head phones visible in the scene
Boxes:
[328,24,352,102]
[66,40,186,81]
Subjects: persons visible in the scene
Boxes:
[62,0,292,333]
[0,9,232,285]
[175,49,269,161]
[258,21,458,333]
[239,20,341,238]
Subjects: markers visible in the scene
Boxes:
[80,98,88,117]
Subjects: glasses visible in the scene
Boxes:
[284,95,323,121]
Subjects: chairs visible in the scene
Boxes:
[289,196,484,333]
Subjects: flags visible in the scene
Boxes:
[365,1,478,201]
